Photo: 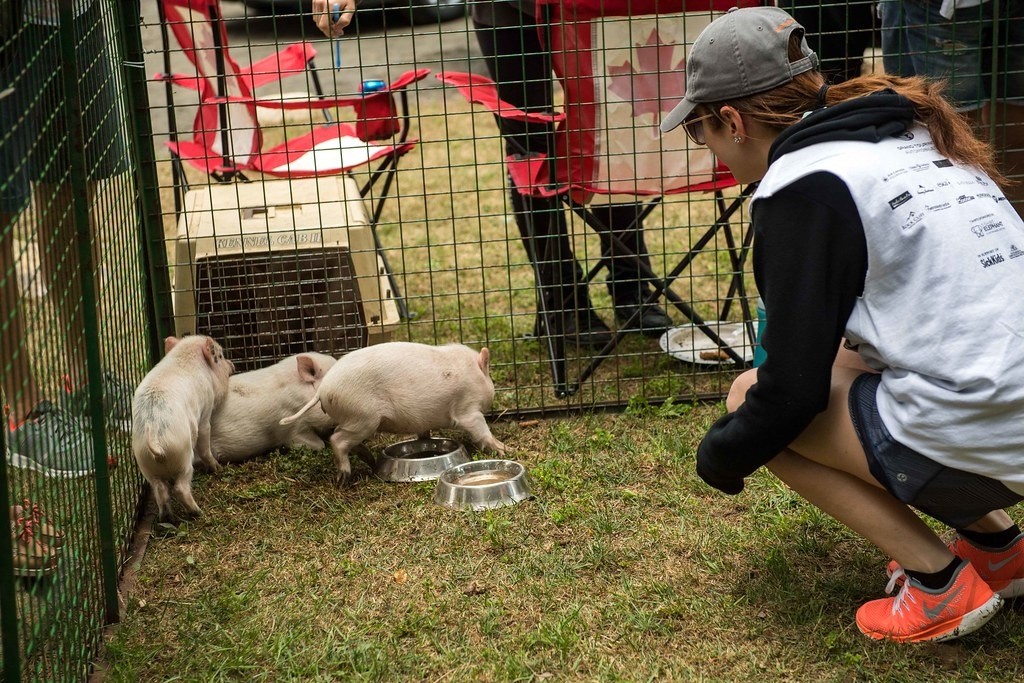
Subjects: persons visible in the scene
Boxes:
[876,0,989,141]
[660,6,1024,645]
[0,0,130,479]
[779,0,873,88]
[312,0,672,351]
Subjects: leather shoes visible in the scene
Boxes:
[541,280,612,350]
[608,276,672,336]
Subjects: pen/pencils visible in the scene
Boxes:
[333,3,341,71]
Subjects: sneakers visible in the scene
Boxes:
[54,369,137,433]
[10,515,57,577]
[4,399,117,480]
[855,558,1005,647]
[9,499,66,549]
[886,529,1024,598]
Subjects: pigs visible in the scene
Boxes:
[190,352,336,466]
[278,341,518,483]
[131,335,235,523]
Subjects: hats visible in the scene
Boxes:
[660,6,820,132]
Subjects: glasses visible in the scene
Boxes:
[681,109,744,145]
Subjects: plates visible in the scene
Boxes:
[659,320,761,364]
